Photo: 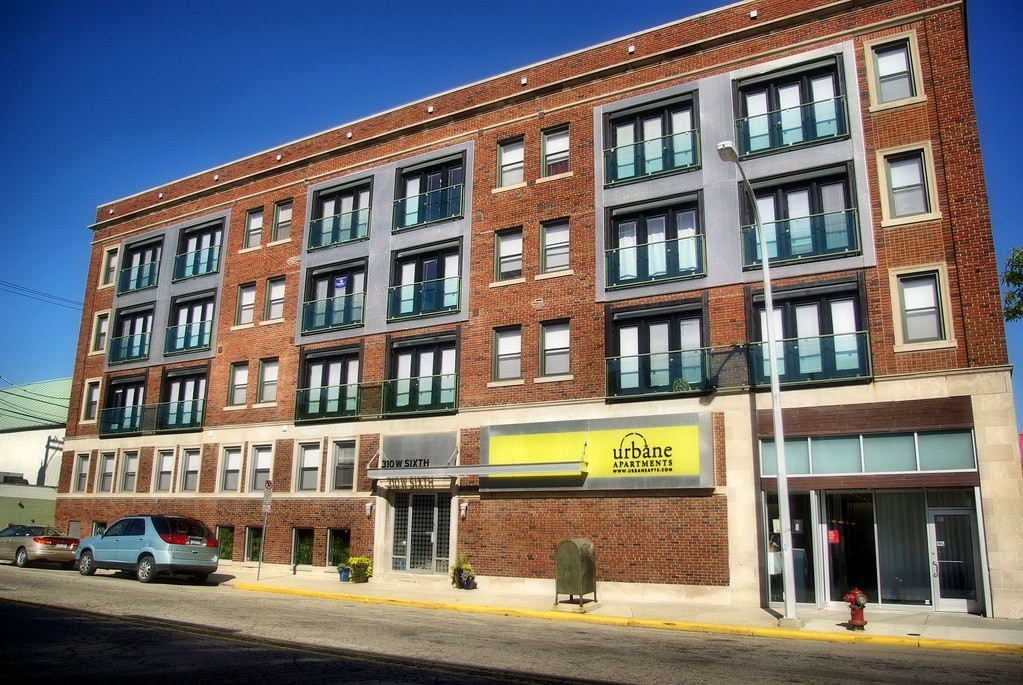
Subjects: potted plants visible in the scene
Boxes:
[337,562,350,582]
[348,556,373,583]
[450,553,477,590]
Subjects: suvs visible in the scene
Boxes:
[74,514,220,583]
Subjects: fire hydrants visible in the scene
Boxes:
[843,588,868,630]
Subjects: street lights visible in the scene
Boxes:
[716,139,798,626]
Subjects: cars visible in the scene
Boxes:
[0,525,81,568]
